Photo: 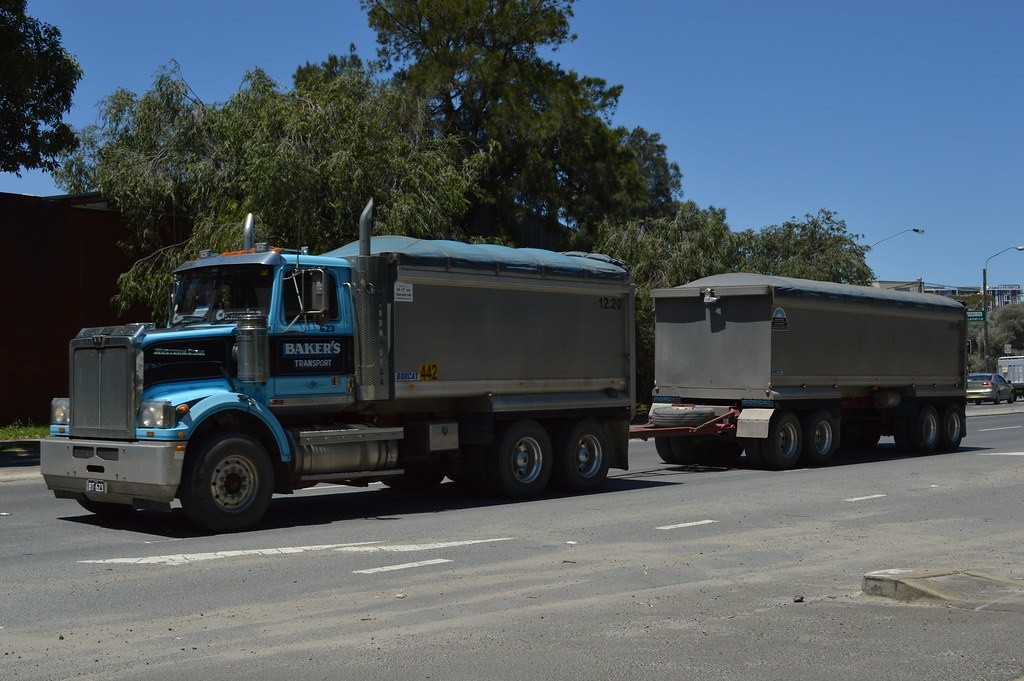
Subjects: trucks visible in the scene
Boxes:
[997,356,1024,402]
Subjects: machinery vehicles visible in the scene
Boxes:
[41,196,636,529]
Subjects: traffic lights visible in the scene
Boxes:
[967,340,971,355]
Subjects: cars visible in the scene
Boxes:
[966,373,1014,405]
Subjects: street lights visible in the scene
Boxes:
[870,228,925,247]
[982,246,1024,373]
